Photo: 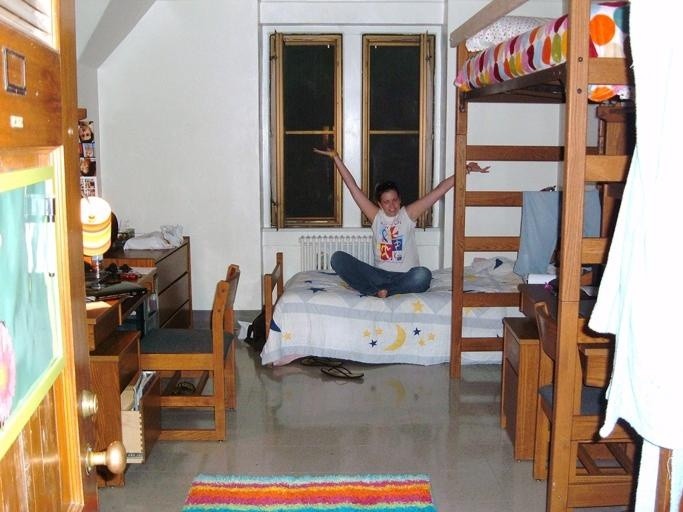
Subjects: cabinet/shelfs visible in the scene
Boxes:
[104,229,194,332]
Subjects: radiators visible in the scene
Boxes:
[298,230,377,274]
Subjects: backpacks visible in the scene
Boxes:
[244,306,266,351]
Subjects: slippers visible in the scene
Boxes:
[321,366,364,378]
[300,356,344,366]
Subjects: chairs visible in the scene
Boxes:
[136,261,243,444]
[529,300,643,512]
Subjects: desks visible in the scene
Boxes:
[81,266,166,490]
[499,272,673,512]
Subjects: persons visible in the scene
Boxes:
[77,121,95,196]
[311,145,491,299]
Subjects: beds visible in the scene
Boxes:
[444,0,674,512]
[255,249,593,372]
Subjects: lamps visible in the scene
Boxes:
[78,195,116,294]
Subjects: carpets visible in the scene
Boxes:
[179,470,441,512]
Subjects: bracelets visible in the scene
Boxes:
[465,165,472,175]
[328,150,337,160]
